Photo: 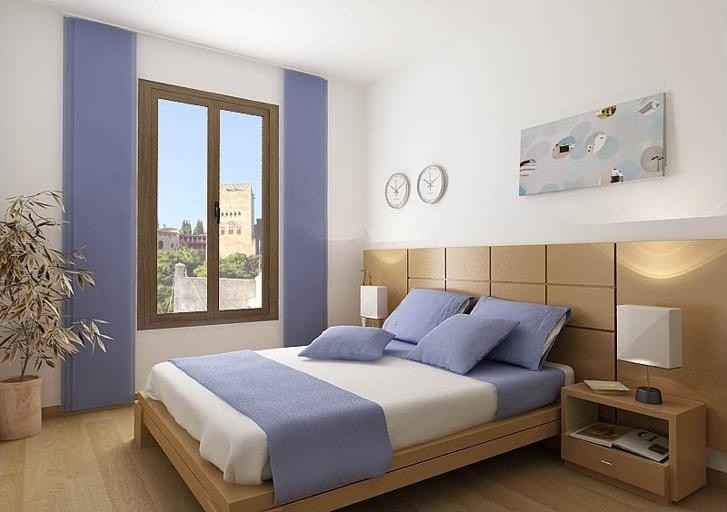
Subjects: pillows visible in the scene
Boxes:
[382,287,474,344]
[471,296,572,371]
[407,313,520,374]
[298,326,396,361]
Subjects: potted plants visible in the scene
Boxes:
[0,190,114,440]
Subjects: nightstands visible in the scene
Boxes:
[560,382,707,506]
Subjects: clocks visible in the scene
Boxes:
[417,165,446,204]
[385,173,410,208]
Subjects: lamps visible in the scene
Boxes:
[360,286,388,327]
[617,305,682,404]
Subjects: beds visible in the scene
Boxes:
[133,340,575,512]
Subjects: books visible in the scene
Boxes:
[567,421,635,449]
[583,380,631,395]
[611,427,670,464]
